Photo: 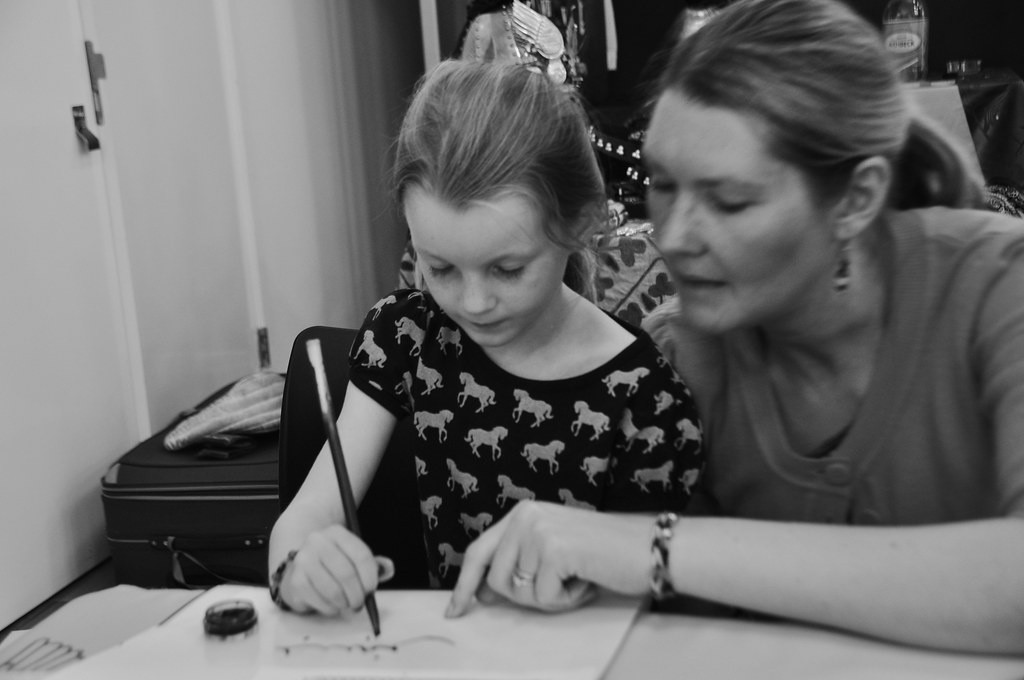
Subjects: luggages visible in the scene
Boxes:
[100,367,281,591]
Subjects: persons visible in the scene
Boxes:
[267,55,706,617]
[437,0,1024,661]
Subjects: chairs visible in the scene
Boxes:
[277,325,430,590]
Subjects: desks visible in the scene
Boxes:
[928,63,1024,195]
[0,580,1024,680]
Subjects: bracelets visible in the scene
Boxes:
[647,509,679,611]
[268,549,317,616]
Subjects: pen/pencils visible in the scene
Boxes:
[305,337,380,635]
